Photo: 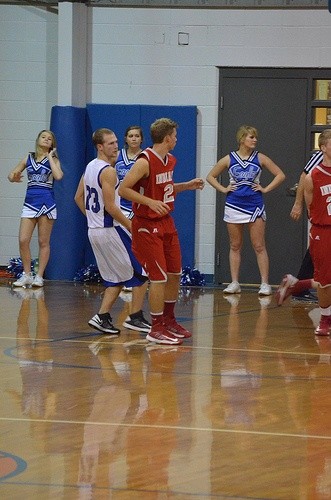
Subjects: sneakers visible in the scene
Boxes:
[291,290,319,304]
[315,315,331,336]
[162,318,192,338]
[223,281,242,294]
[87,312,121,334]
[12,272,34,286]
[146,330,183,345]
[122,310,152,333]
[31,274,44,287]
[258,283,272,296]
[276,274,298,306]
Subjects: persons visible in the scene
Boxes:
[7,128,64,288]
[76,332,181,500]
[273,304,331,500]
[73,117,205,346]
[11,285,59,439]
[276,128,331,334]
[201,296,277,462]
[207,124,287,295]
